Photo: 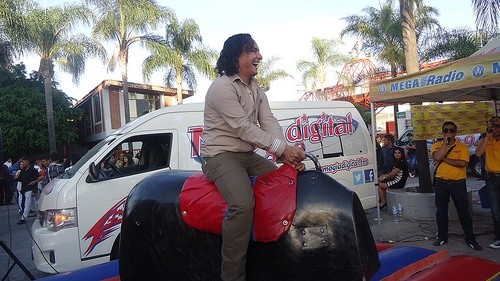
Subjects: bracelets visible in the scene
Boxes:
[382,176,385,179]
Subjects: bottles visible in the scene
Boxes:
[397,201,404,220]
[392,204,400,224]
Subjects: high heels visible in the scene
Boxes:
[377,202,387,211]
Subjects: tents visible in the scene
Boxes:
[369,34,500,242]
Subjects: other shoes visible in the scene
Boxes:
[28,211,36,217]
[17,220,26,225]
[408,173,416,178]
[466,239,482,251]
[489,240,500,249]
[433,238,448,246]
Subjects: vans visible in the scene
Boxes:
[32,100,380,276]
[396,127,482,178]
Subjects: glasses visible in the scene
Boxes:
[443,128,455,133]
[490,124,500,129]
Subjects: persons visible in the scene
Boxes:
[115,151,140,170]
[432,122,483,251]
[477,133,486,180]
[476,116,500,249]
[199,34,306,281]
[375,133,418,210]
[0,152,74,225]
